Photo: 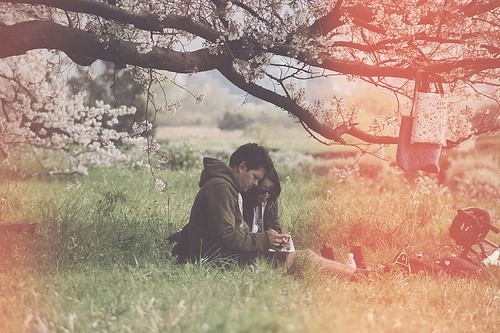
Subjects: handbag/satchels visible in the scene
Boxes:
[396,69,448,173]
[410,69,450,146]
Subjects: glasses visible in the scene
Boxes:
[257,185,276,194]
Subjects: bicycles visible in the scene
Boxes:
[430,207,500,276]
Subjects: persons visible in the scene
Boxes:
[176,140,388,284]
[236,161,292,252]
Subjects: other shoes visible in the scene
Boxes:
[352,269,380,282]
